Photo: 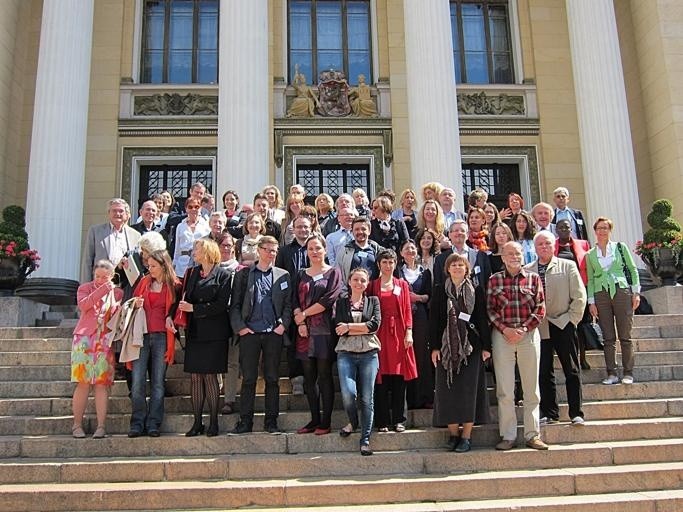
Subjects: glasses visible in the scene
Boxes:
[304,212,316,218]
[261,247,277,255]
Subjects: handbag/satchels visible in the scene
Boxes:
[174,303,187,327]
[623,266,633,285]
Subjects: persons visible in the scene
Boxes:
[348,74,377,117]
[286,73,321,117]
[69,182,592,458]
[584,217,641,385]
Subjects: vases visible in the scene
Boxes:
[0,257,36,297]
[640,248,683,286]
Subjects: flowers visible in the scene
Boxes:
[632,199,682,256]
[0,205,42,271]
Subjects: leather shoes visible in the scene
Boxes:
[581,360,592,369]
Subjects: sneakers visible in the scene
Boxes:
[602,377,618,385]
[622,375,634,385]
[292,376,305,396]
[222,401,234,414]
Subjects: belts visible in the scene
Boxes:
[182,250,191,255]
